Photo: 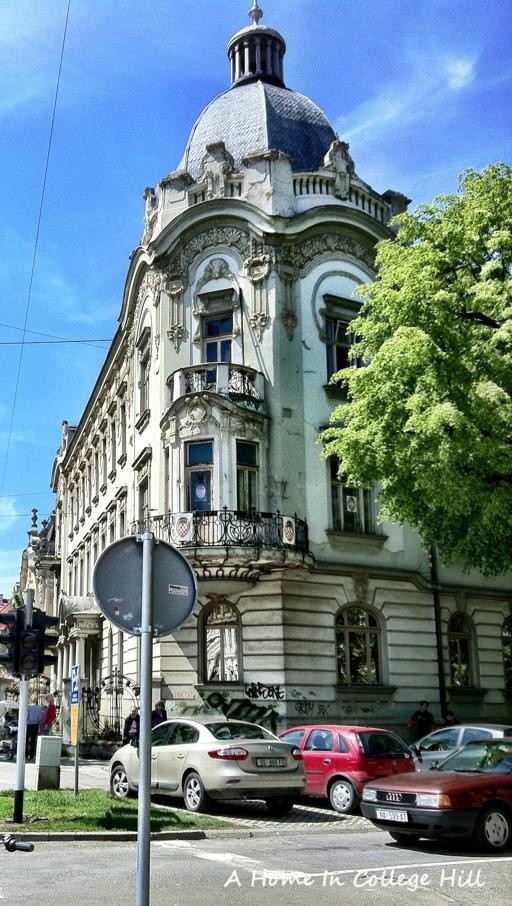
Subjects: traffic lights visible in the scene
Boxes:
[35,609,60,674]
[20,630,41,675]
[0,609,24,676]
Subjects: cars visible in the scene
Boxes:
[407,723,512,772]
[277,724,416,814]
[109,715,306,815]
[360,737,512,854]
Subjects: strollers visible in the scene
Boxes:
[4,720,34,763]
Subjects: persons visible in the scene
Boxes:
[1,691,59,763]
[123,706,140,748]
[406,700,436,744]
[485,749,504,771]
[150,699,167,730]
[441,709,461,728]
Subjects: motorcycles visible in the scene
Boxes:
[0,833,34,852]
